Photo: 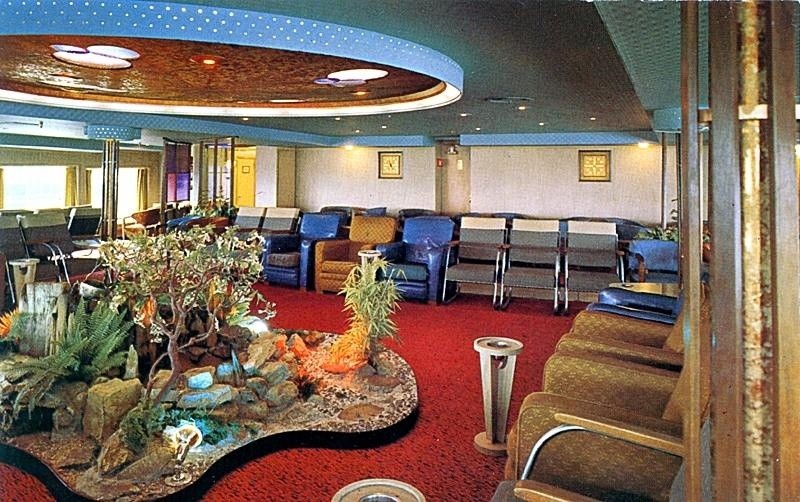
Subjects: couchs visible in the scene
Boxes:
[484,392,714,502]
[318,207,644,280]
[501,325,711,478]
[374,216,456,306]
[315,216,396,297]
[261,211,347,292]
[232,207,304,282]
[551,276,714,375]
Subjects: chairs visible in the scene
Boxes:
[123,203,229,244]
[498,219,560,313]
[1,204,114,310]
[626,240,679,283]
[561,220,625,316]
[440,217,508,315]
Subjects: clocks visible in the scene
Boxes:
[378,151,403,179]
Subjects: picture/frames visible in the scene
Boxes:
[578,150,612,182]
